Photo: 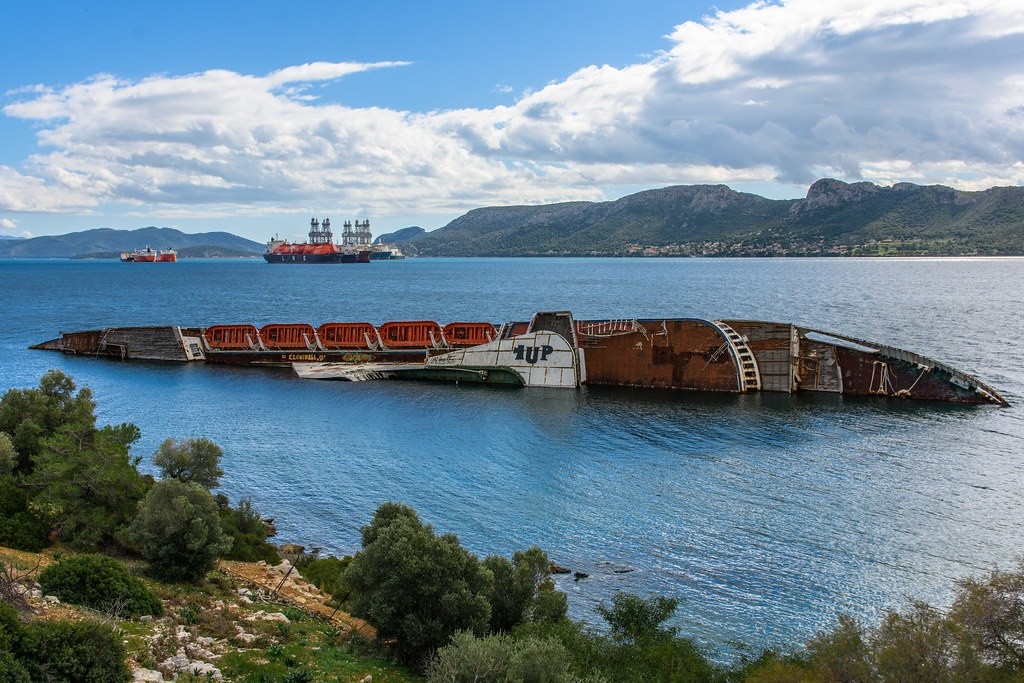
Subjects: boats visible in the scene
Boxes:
[120,244,178,263]
[335,219,404,260]
[264,216,372,262]
